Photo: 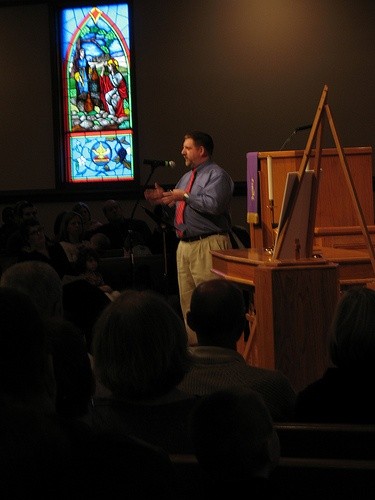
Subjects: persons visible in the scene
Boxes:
[143,131,234,365]
[173,389,312,500]
[0,200,202,445]
[289,287,375,500]
[176,281,297,421]
[0,286,173,500]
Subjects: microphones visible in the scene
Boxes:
[141,158,176,168]
[294,123,313,132]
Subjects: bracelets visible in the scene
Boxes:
[182,193,189,201]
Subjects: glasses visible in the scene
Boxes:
[28,227,43,236]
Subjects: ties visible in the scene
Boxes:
[174,167,198,237]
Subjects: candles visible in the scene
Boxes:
[266,154,274,200]
